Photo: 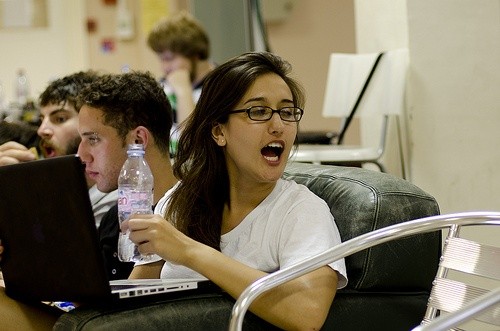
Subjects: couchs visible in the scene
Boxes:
[50,165,441,330]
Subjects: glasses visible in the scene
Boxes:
[230,105,304,123]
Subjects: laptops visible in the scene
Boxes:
[0,154,225,314]
[293,52,383,145]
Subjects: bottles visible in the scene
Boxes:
[117,144,154,261]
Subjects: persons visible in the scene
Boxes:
[0,70,188,331]
[147,13,221,163]
[120,50,348,331]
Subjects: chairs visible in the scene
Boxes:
[289,51,408,172]
[231,212,499,327]
